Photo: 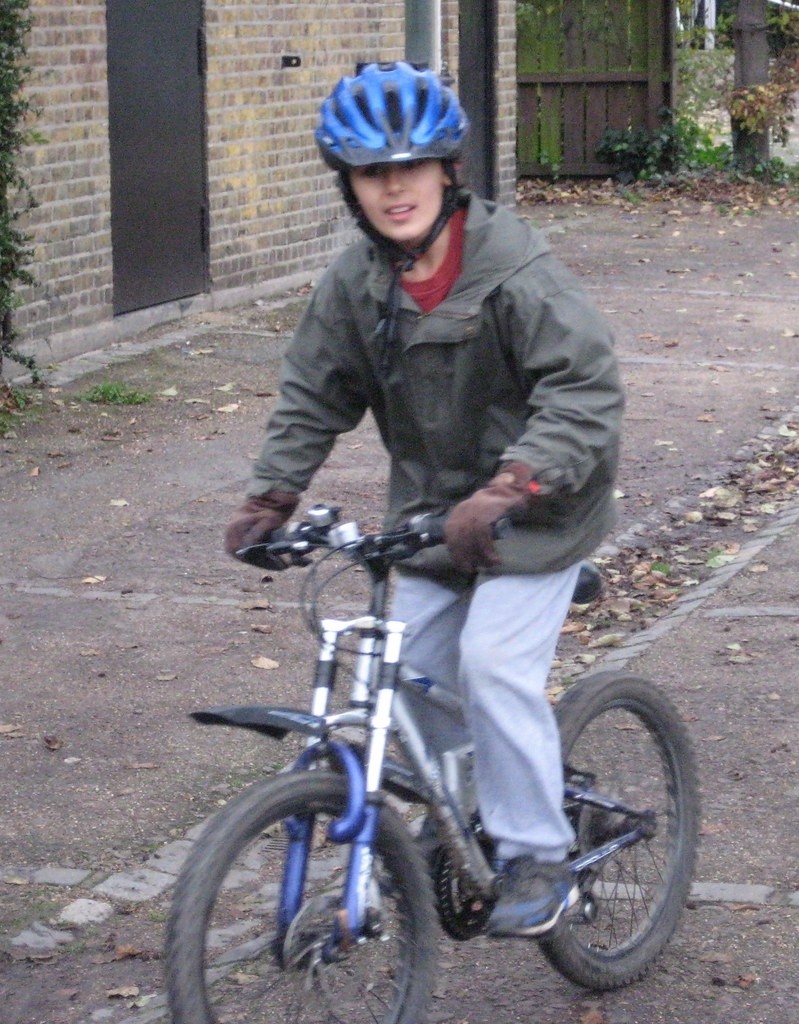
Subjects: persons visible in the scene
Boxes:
[226,61,626,939]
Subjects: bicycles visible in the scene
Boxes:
[162,497,703,1022]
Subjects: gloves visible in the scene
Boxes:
[440,462,536,571]
[221,487,306,573]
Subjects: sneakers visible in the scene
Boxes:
[480,853,582,938]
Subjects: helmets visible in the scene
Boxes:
[317,57,474,171]
